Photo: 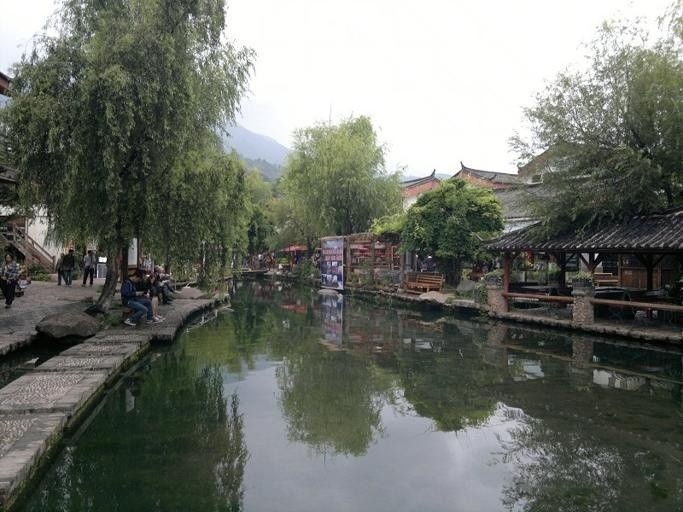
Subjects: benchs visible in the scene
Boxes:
[121,307,134,321]
[401,271,445,293]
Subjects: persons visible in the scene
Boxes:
[53,248,177,327]
[0,252,19,309]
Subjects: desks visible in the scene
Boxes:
[522,280,683,322]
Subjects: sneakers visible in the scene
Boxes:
[57,283,72,288]
[81,284,92,287]
[123,318,137,326]
[145,314,166,325]
[5,301,11,309]
